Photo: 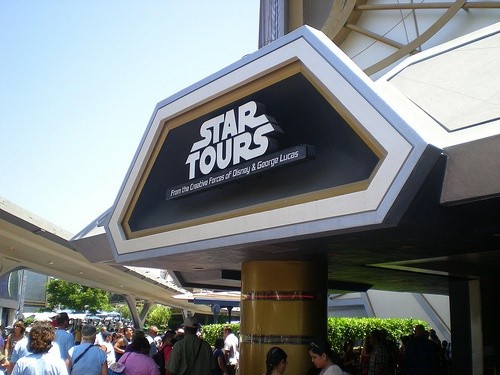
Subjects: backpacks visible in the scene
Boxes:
[108,351,132,375]
[153,344,171,375]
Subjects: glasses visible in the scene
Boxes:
[311,342,321,349]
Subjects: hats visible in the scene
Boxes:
[102,331,111,339]
[82,325,99,338]
[180,317,199,328]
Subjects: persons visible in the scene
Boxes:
[159,317,239,375]
[343,340,361,375]
[0,312,161,375]
[361,324,450,375]
[308,339,351,375]
[263,347,288,375]
